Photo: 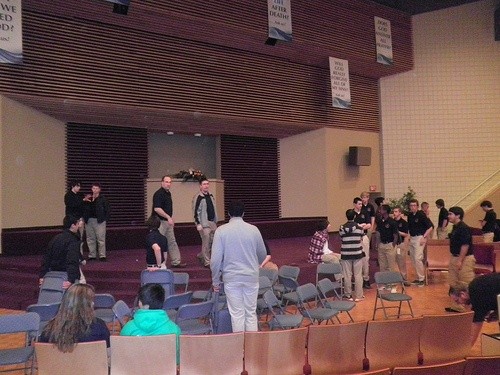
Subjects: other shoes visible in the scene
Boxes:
[342,295,353,301]
[364,281,371,288]
[171,262,187,268]
[100,257,106,262]
[355,295,366,301]
[404,282,411,286]
[412,280,419,284]
[418,281,425,287]
[445,307,458,312]
[86,257,95,261]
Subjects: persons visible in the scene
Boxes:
[36,174,188,365]
[259,239,281,298]
[209,200,267,332]
[192,177,218,267]
[307,192,500,350]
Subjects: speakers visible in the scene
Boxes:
[348,146,372,166]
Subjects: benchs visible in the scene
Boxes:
[425,239,452,286]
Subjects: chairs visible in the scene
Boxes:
[365,320,419,372]
[372,271,416,319]
[0,263,369,375]
[420,311,475,364]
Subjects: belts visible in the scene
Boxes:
[147,265,156,267]
[383,240,393,244]
